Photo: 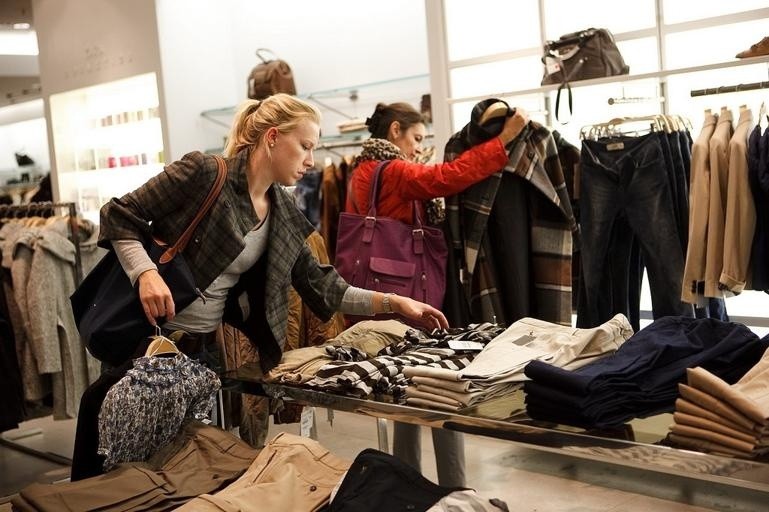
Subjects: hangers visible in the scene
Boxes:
[0,202,89,233]
[476,93,511,129]
[292,134,434,171]
[141,312,182,361]
[569,82,768,142]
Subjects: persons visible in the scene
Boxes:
[344,102,533,490]
[70,92,451,482]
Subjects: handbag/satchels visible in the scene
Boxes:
[245,48,297,100]
[68,152,227,365]
[538,27,631,132]
[336,160,447,324]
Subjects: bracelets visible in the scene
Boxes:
[382,291,398,315]
